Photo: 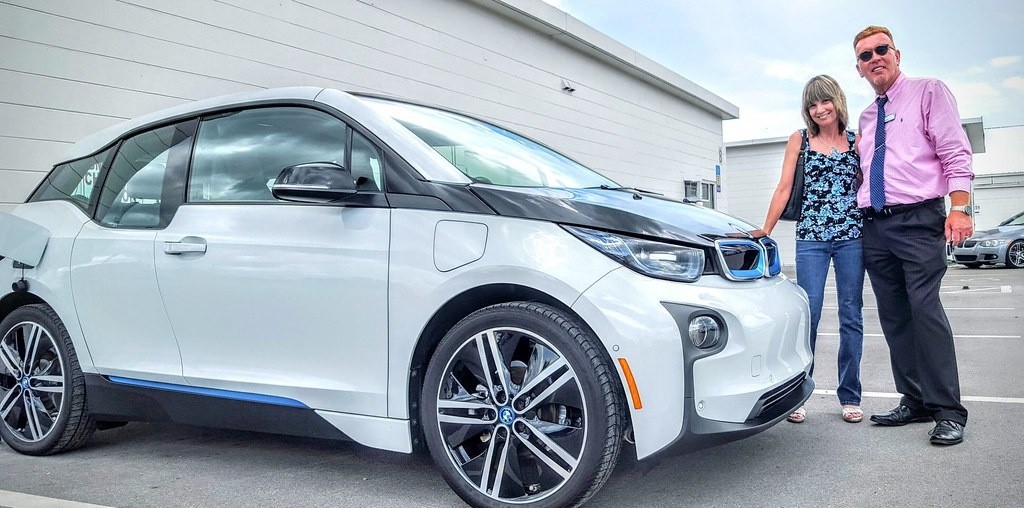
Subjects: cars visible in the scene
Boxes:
[0,86,815,508]
[953,211,1024,269]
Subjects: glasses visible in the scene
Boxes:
[857,44,896,63]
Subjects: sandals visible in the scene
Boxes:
[787,407,806,423]
[842,408,864,422]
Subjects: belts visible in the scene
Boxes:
[861,197,944,217]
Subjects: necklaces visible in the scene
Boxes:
[818,130,836,148]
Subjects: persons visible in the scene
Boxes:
[764,75,864,423]
[853,25,975,445]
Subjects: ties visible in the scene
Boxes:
[870,95,888,213]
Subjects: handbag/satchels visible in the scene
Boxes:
[779,129,806,221]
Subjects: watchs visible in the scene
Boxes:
[950,206,972,215]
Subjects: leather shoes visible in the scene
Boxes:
[870,405,934,426]
[928,419,964,444]
